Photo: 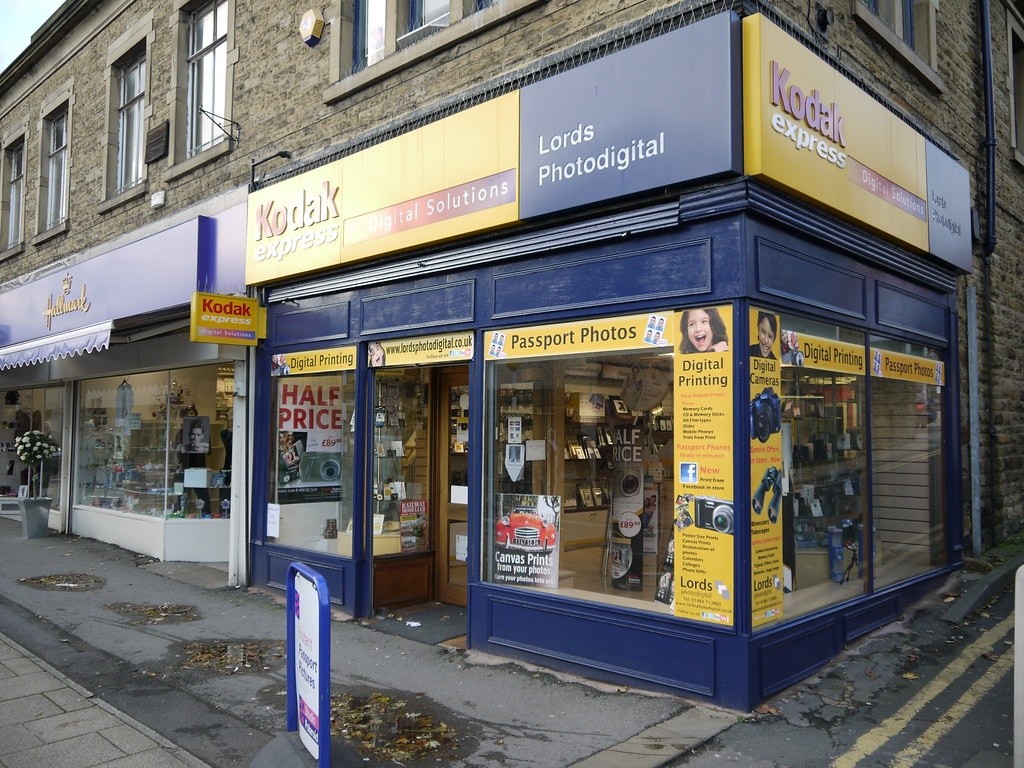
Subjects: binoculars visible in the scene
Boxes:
[752,466,783,525]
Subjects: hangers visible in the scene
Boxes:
[120,376,128,386]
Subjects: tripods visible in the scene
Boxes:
[840,549,861,586]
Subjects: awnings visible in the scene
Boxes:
[0,319,112,372]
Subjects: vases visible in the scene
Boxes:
[19,495,53,539]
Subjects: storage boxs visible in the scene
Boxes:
[184,468,214,488]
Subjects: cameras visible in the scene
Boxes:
[750,386,781,443]
[694,496,735,535]
[299,451,342,482]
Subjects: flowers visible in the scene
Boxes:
[14,430,59,464]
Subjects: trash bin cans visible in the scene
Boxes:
[16,497,53,539]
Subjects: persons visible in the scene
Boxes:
[782,330,791,354]
[490,332,504,356]
[679,309,728,353]
[286,449,299,464]
[749,310,778,360]
[644,317,664,344]
[673,494,693,527]
[368,342,386,367]
[272,354,280,371]
[183,420,209,453]
[643,495,656,529]
[173,404,212,519]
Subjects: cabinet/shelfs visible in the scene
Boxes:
[77,361,864,593]
